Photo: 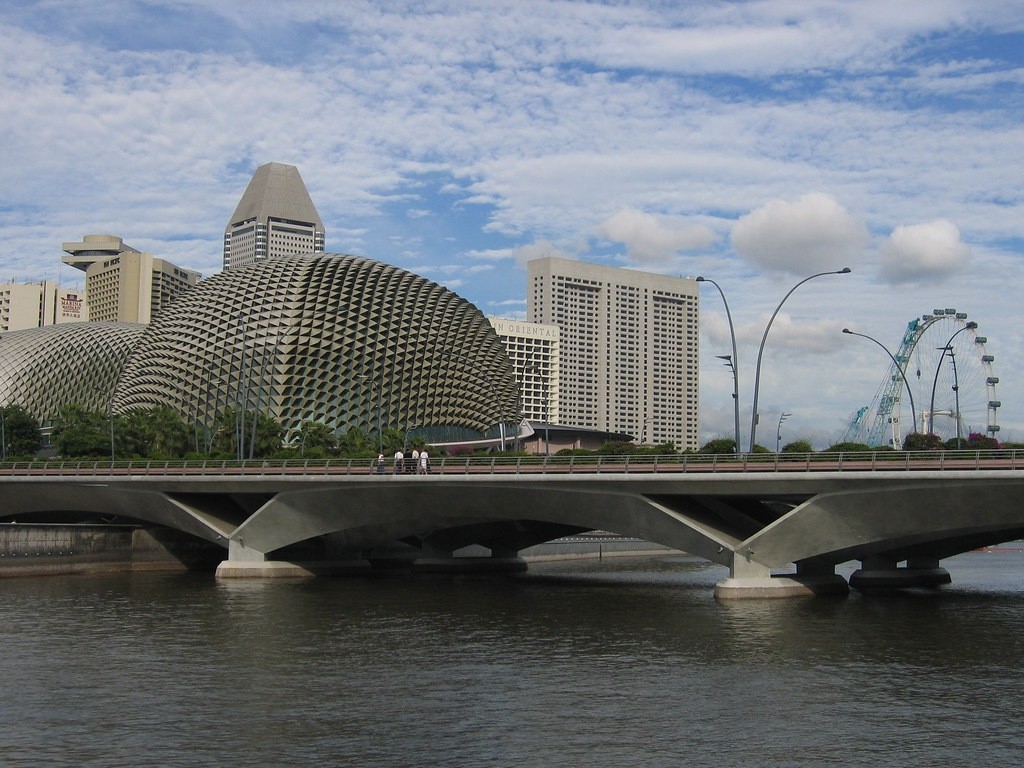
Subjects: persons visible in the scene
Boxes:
[411,449,419,475]
[394,449,403,474]
[404,449,412,474]
[377,452,385,475]
[420,449,429,475]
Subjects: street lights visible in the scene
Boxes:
[930,322,978,436]
[750,266,851,453]
[696,276,741,462]
[529,365,549,455]
[512,347,541,452]
[93,387,114,462]
[356,373,384,459]
[842,327,917,434]
[249,306,347,460]
[237,314,246,459]
[440,350,506,453]
